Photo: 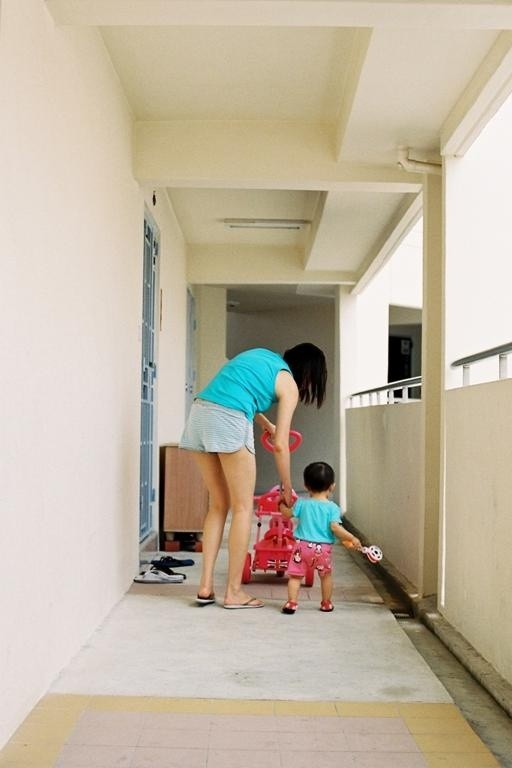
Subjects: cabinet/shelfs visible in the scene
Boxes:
[161,438,218,553]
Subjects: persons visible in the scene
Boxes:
[279,461,363,612]
[178,342,328,609]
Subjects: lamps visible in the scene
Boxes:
[222,217,312,233]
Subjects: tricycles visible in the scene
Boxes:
[243,428,315,588]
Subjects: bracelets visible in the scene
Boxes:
[278,498,284,512]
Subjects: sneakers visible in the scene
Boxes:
[320,600,333,611]
[283,600,297,613]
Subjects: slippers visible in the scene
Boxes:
[197,592,216,603]
[134,556,194,583]
[224,598,262,609]
